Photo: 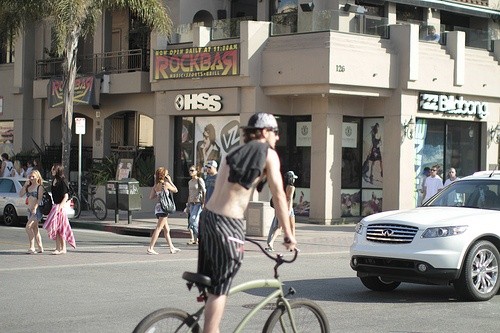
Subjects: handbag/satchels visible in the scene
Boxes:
[160,182,176,214]
[37,184,52,215]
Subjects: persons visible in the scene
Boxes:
[145,168,182,255]
[180,124,223,171]
[421,166,460,207]
[199,113,297,333]
[184,161,218,245]
[264,172,302,253]
[0,153,76,255]
[343,123,385,216]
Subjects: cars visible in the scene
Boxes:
[0,177,76,226]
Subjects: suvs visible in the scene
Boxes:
[349,171,500,301]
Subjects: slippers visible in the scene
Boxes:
[265,247,275,251]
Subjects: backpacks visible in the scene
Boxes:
[68,180,74,199]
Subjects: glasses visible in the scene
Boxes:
[202,133,209,140]
[267,127,279,136]
[190,170,195,172]
[430,169,436,171]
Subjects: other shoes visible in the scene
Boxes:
[51,249,66,255]
[146,249,159,254]
[186,239,195,244]
[25,248,46,254]
[169,248,182,253]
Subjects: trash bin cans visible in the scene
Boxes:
[105,180,118,210]
[118,177,142,211]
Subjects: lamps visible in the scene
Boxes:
[344,3,368,14]
[300,2,314,12]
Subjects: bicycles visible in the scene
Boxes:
[134,237,331,333]
[69,188,108,220]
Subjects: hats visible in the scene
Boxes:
[205,160,217,168]
[239,113,278,130]
[286,171,298,178]
